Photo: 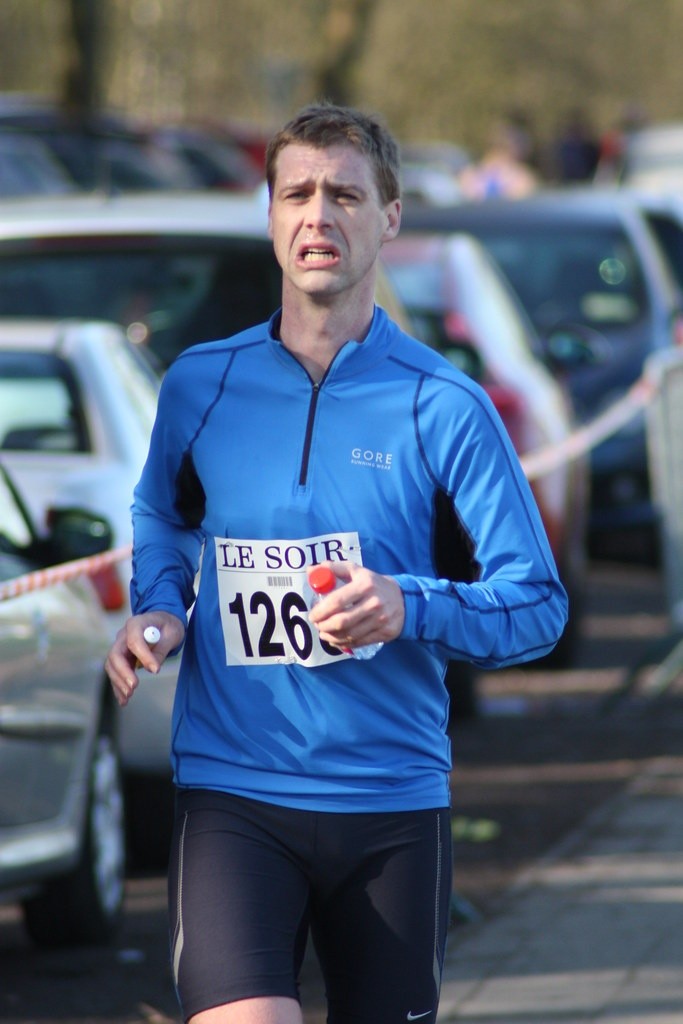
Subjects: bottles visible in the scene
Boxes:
[310,568,384,660]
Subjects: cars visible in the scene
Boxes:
[0,312,187,782]
[0,455,131,944]
[0,99,683,722]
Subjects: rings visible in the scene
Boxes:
[346,635,355,643]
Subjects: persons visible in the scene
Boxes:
[102,100,570,1024]
